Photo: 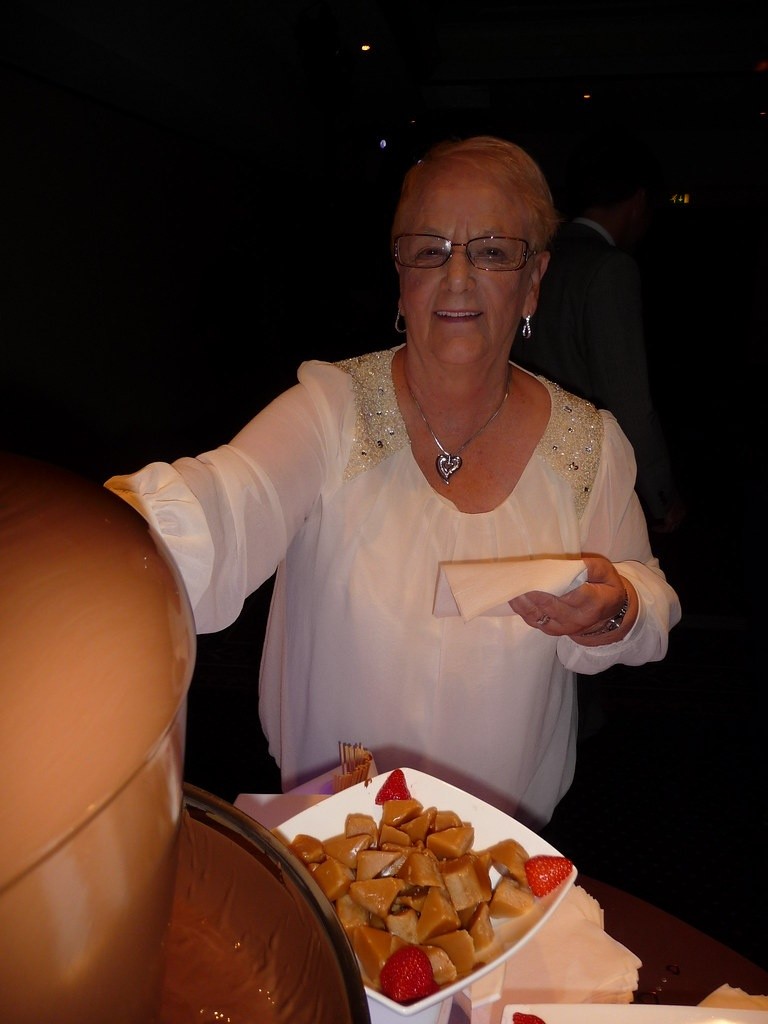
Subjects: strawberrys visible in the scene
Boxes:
[374,769,410,805]
[525,854,573,898]
[378,945,439,1005]
[512,1012,546,1024]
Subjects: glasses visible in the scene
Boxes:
[393,232,540,271]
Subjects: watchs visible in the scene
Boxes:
[582,588,629,637]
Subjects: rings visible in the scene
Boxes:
[537,615,550,624]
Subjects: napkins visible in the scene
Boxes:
[696,982,768,1011]
[462,884,642,1024]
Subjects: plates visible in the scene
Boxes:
[270,767,577,1016]
[500,1003,767,1024]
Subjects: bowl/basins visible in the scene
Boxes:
[155,782,372,1024]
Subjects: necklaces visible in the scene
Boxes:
[410,373,510,484]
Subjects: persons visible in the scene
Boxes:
[103,136,680,880]
[519,176,689,534]
